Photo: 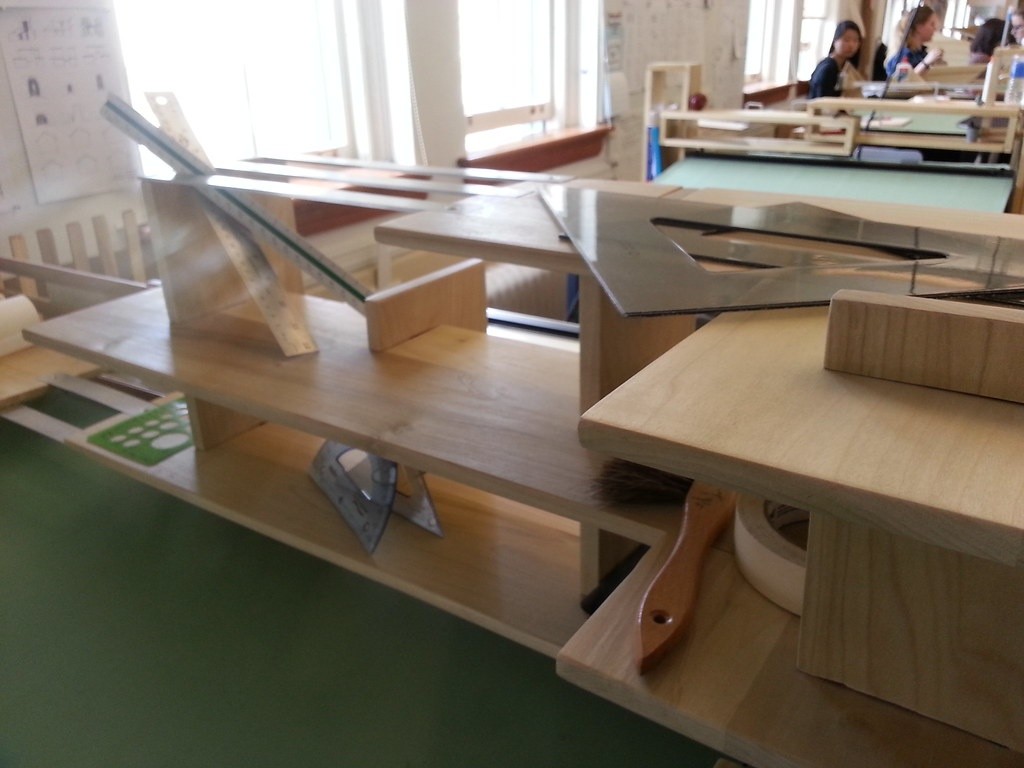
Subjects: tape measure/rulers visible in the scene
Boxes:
[144,86,323,358]
[300,435,446,558]
[533,180,1024,324]
[99,93,375,322]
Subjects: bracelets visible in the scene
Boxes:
[921,60,930,69]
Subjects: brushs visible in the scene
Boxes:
[632,479,740,673]
[585,455,691,511]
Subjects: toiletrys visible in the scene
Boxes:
[894,56,915,88]
[980,53,1003,106]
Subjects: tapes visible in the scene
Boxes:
[737,486,807,619]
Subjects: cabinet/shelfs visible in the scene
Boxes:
[18,157,1024,768]
[640,27,1024,212]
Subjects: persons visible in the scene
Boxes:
[804,18,862,103]
[1009,14,1024,46]
[885,4,946,81]
[967,18,1013,67]
